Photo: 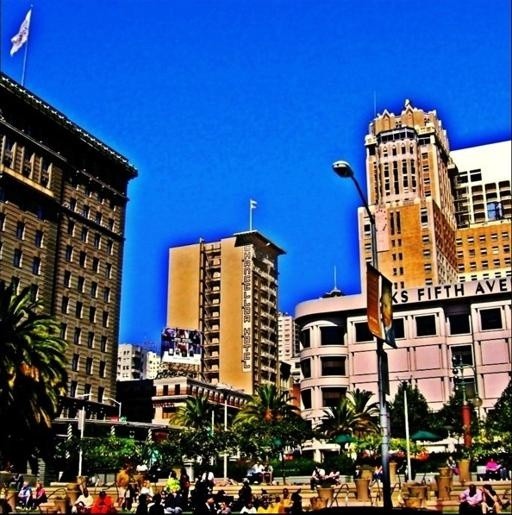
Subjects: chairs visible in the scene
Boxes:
[310,461,452,509]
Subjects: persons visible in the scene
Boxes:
[381,287,394,347]
[0,450,512,515]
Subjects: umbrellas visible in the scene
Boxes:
[410,430,441,450]
[327,433,359,445]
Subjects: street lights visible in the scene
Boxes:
[332,160,393,511]
